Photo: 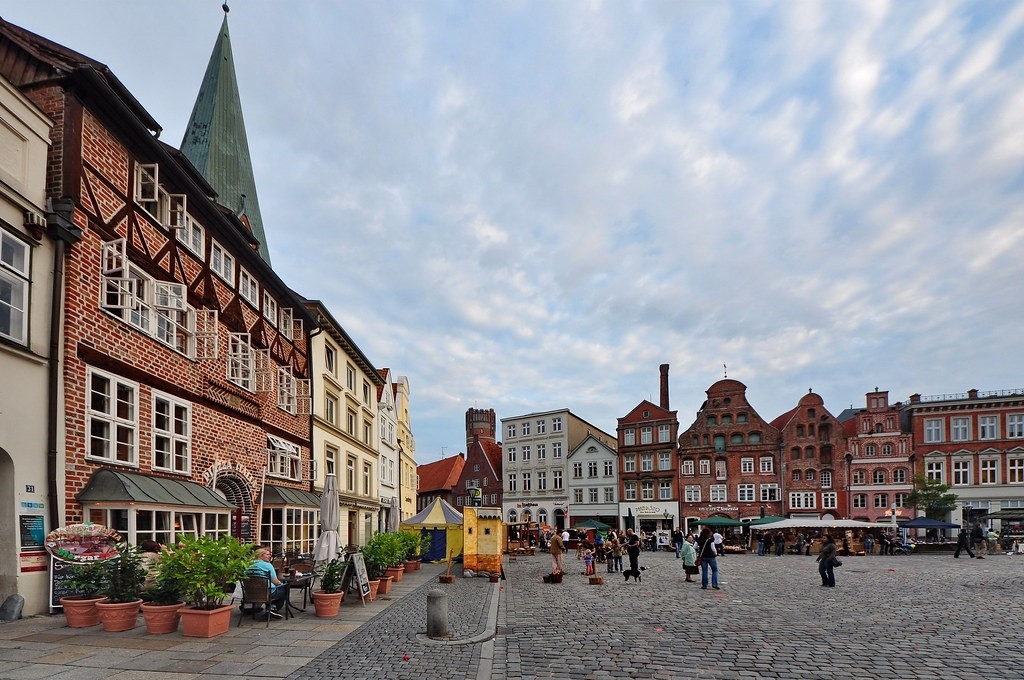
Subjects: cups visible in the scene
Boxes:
[289,570,296,579]
[285,567,289,574]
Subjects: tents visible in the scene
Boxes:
[748,519,898,549]
[900,516,962,543]
[399,496,463,563]
[574,519,611,532]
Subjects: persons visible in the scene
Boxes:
[621,528,639,571]
[816,534,836,587]
[511,525,570,554]
[134,540,198,591]
[605,539,623,573]
[582,539,596,575]
[694,528,720,590]
[680,534,700,582]
[673,527,731,557]
[843,529,895,556]
[242,545,286,619]
[973,523,986,559]
[756,529,814,556]
[987,528,999,555]
[550,529,568,574]
[594,529,627,562]
[954,529,976,558]
[906,535,914,543]
[641,529,657,552]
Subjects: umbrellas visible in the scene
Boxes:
[311,473,346,571]
[387,496,399,532]
[689,515,786,539]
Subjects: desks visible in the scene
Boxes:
[567,538,581,549]
[274,573,319,620]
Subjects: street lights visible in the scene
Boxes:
[466,481,479,507]
[663,510,669,530]
[962,501,974,550]
[845,453,853,520]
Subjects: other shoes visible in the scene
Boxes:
[828,584,835,587]
[820,583,828,586]
[586,573,589,576]
[562,572,566,575]
[685,578,695,582]
[701,586,707,589]
[712,585,720,590]
[265,608,283,620]
[589,572,593,575]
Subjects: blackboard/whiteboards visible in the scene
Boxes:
[50,557,121,607]
[657,529,671,546]
[20,515,45,548]
[338,553,371,596]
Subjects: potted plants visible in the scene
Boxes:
[60,530,256,638]
[312,529,433,616]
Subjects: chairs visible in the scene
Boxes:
[281,554,303,575]
[237,574,276,627]
[287,559,316,609]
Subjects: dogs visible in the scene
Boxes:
[623,566,647,584]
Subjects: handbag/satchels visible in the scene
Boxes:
[695,557,701,566]
[830,557,841,567]
